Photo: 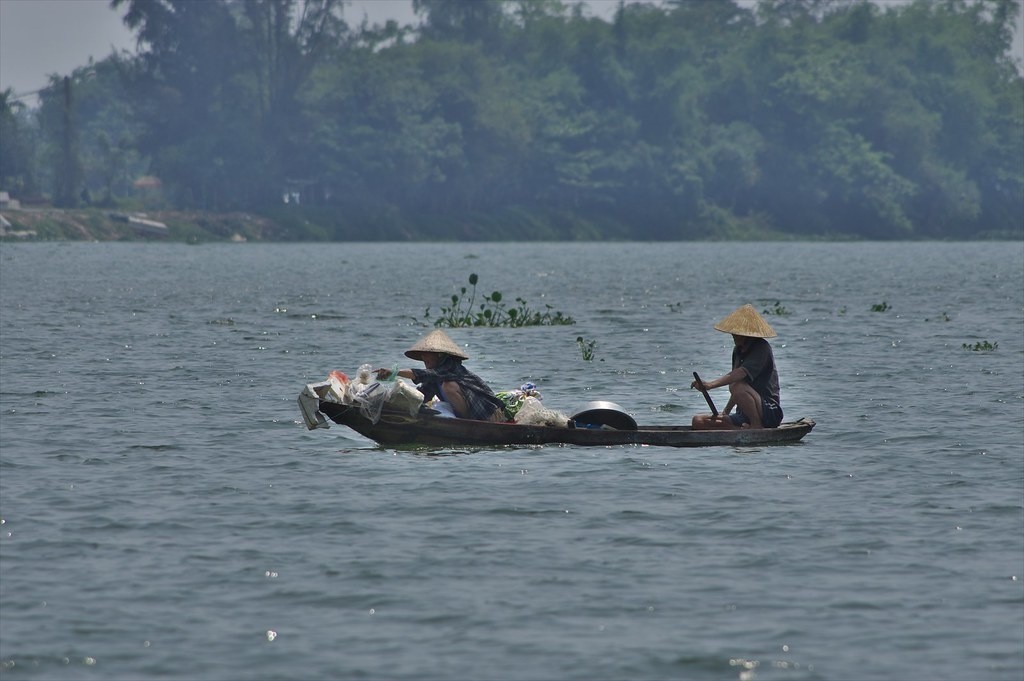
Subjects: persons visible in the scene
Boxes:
[691,304,784,430]
[370,328,506,421]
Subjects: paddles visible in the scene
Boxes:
[693,371,721,416]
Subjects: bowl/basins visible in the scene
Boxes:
[569,401,639,431]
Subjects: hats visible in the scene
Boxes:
[714,304,777,338]
[404,330,469,360]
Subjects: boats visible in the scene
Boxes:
[295,371,817,447]
